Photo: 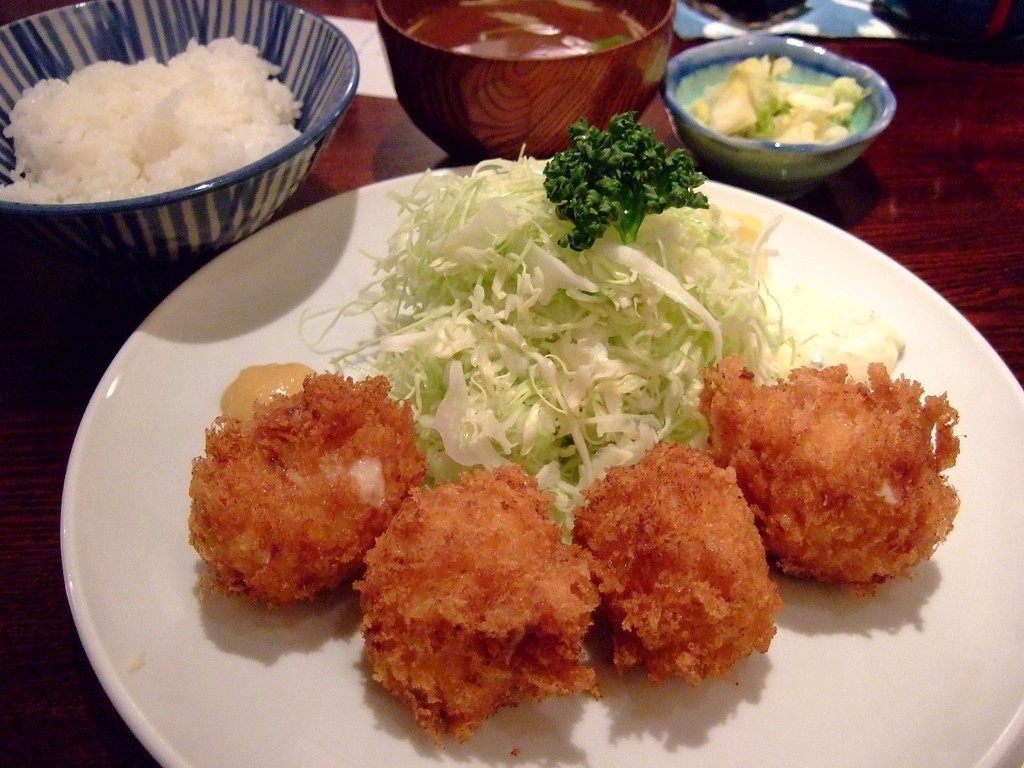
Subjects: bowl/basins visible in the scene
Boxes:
[374,1,678,163]
[658,35,897,202]
[0,0,358,281]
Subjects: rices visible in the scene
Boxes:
[0,35,304,210]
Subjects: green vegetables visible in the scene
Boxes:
[542,113,711,252]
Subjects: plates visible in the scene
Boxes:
[60,161,1024,768]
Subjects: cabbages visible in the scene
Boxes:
[325,147,900,539]
